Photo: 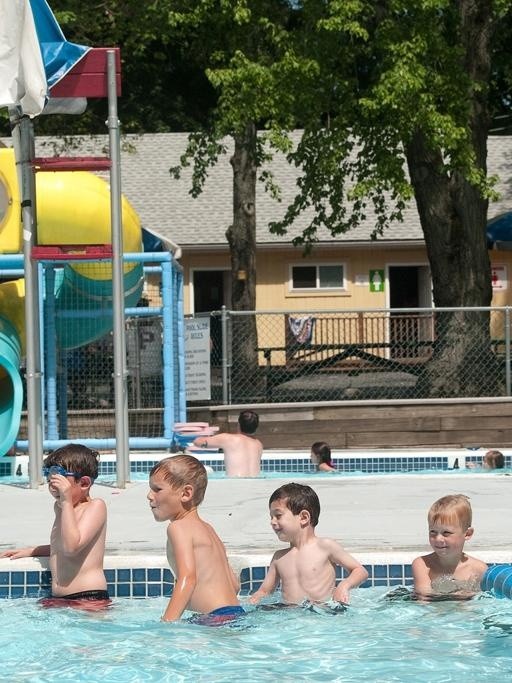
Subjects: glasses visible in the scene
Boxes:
[41,466,79,477]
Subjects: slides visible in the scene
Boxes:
[1,148,145,462]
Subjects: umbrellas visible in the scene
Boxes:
[486,210,511,251]
[0,1,93,219]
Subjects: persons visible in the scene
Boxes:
[466,450,506,470]
[193,410,263,477]
[245,482,368,604]
[1,443,111,618]
[147,455,255,627]
[410,493,490,598]
[310,441,337,470]
